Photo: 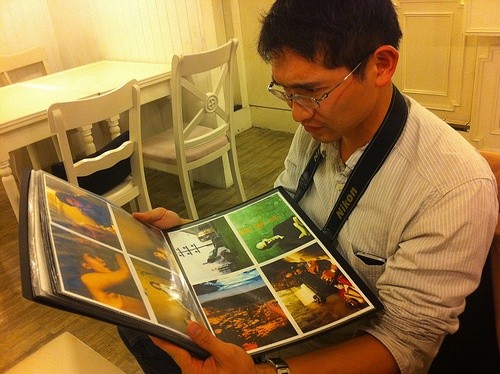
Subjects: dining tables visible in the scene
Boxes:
[0,59,171,222]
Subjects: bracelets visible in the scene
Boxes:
[267,358,291,374]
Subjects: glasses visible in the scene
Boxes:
[268,48,379,112]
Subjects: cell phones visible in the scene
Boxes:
[356,252,385,266]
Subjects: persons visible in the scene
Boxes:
[43,176,374,358]
[118,0,498,374]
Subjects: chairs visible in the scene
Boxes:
[0,47,82,180]
[47,79,153,216]
[142,37,238,221]
